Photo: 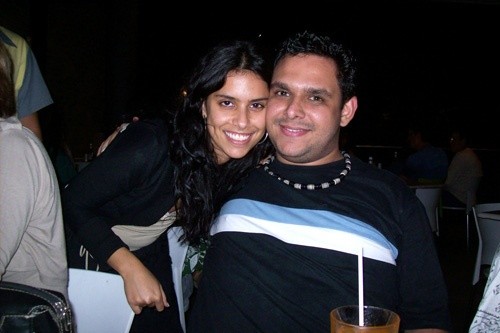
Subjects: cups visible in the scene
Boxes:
[329,304,400,333]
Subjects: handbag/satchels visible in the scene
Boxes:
[0,281,73,333]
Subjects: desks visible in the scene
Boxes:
[353,145,405,167]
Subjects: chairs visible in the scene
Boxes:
[444,178,476,232]
[67,267,136,333]
[472,200,500,286]
[410,183,442,237]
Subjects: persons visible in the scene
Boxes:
[0,42,77,333]
[98,30,448,333]
[0,26,54,143]
[400,126,484,209]
[59,39,269,333]
